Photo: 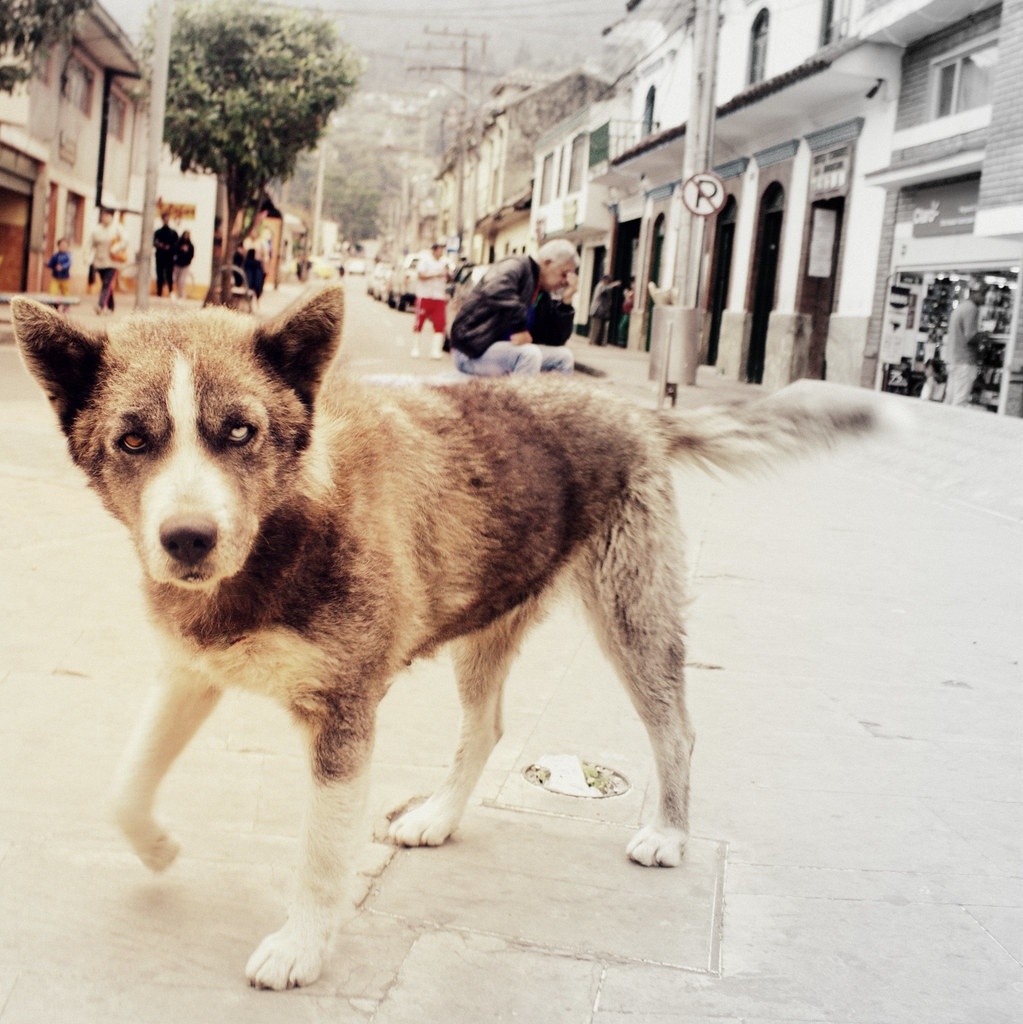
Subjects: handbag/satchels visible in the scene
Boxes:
[111,224,126,262]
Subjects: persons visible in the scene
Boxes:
[153,213,178,296]
[448,237,581,378]
[618,277,635,347]
[942,280,990,406]
[411,241,457,360]
[243,248,266,299]
[589,276,620,346]
[43,238,71,312]
[92,208,127,315]
[232,243,245,287]
[172,230,195,297]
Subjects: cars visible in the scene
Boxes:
[366,253,496,352]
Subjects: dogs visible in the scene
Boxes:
[8,280,884,991]
